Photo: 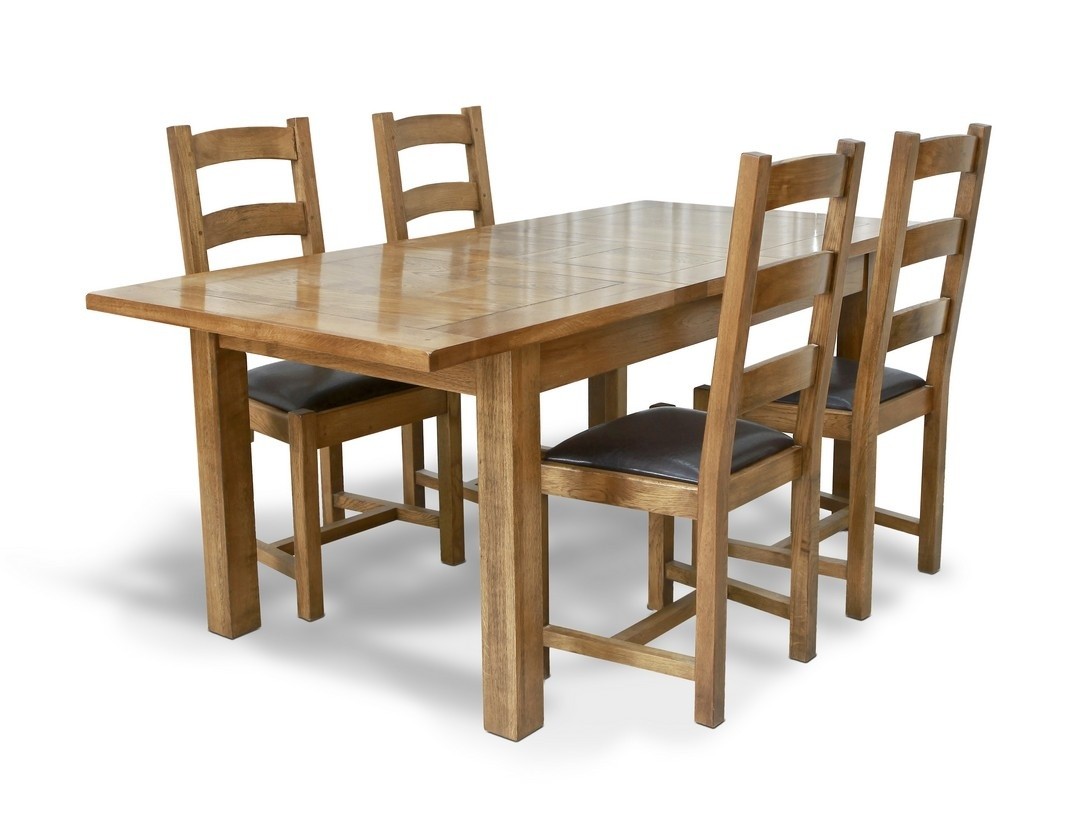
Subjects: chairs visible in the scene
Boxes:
[538,138,865,731]
[694,123,990,622]
[165,116,468,624]
[370,105,550,508]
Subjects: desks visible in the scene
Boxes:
[85,200,927,742]
